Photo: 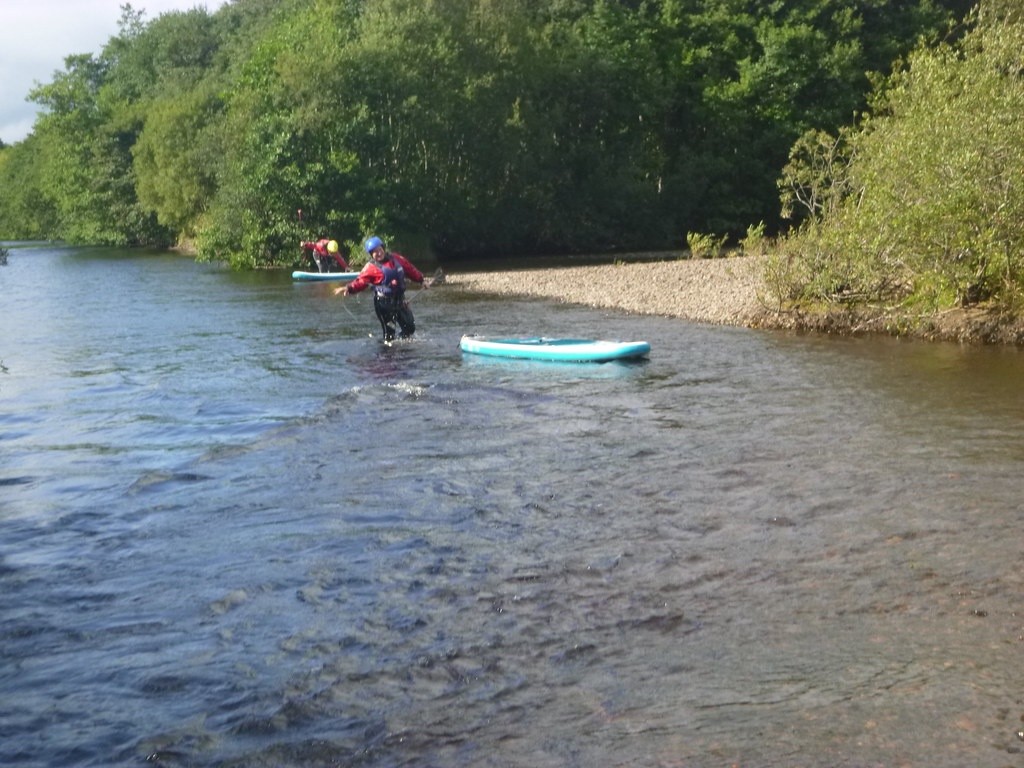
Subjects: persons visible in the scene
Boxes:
[300,238,351,273]
[332,236,430,341]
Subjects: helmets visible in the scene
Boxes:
[366,237,385,257]
[327,240,338,252]
